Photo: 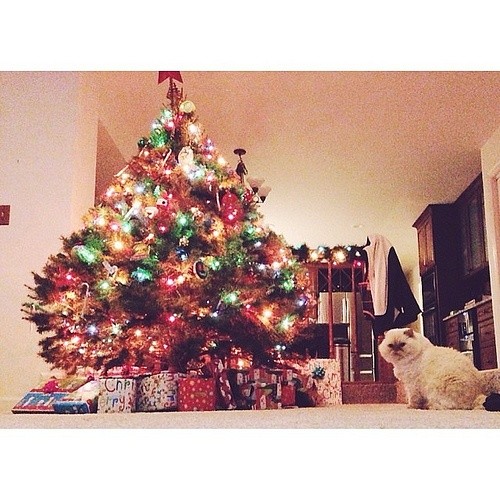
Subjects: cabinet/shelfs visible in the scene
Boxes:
[442,299,498,371]
[454,180,489,284]
[421,309,441,347]
[417,216,435,278]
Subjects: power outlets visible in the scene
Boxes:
[0,205,11,227]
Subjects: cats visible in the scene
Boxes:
[377,328,500,410]
